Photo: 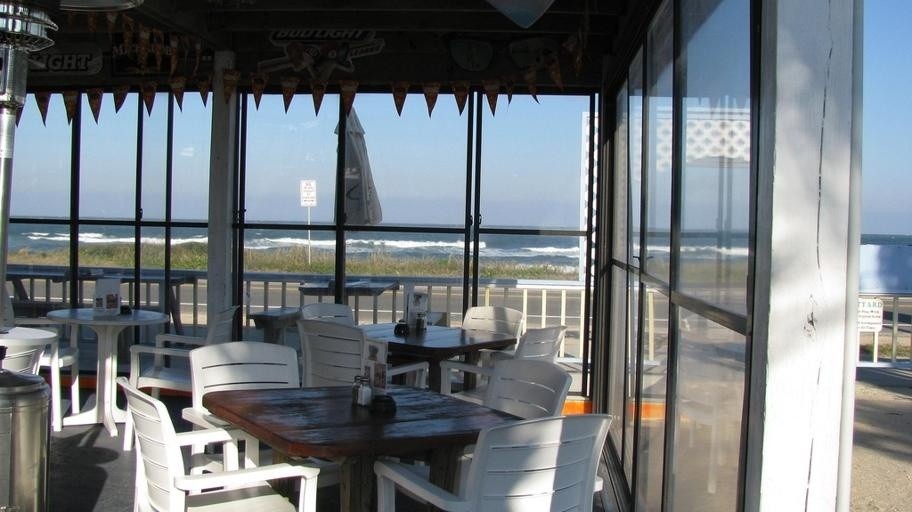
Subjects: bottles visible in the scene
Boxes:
[358,378,372,406]
[416,314,425,329]
[352,376,362,403]
[427,311,432,325]
